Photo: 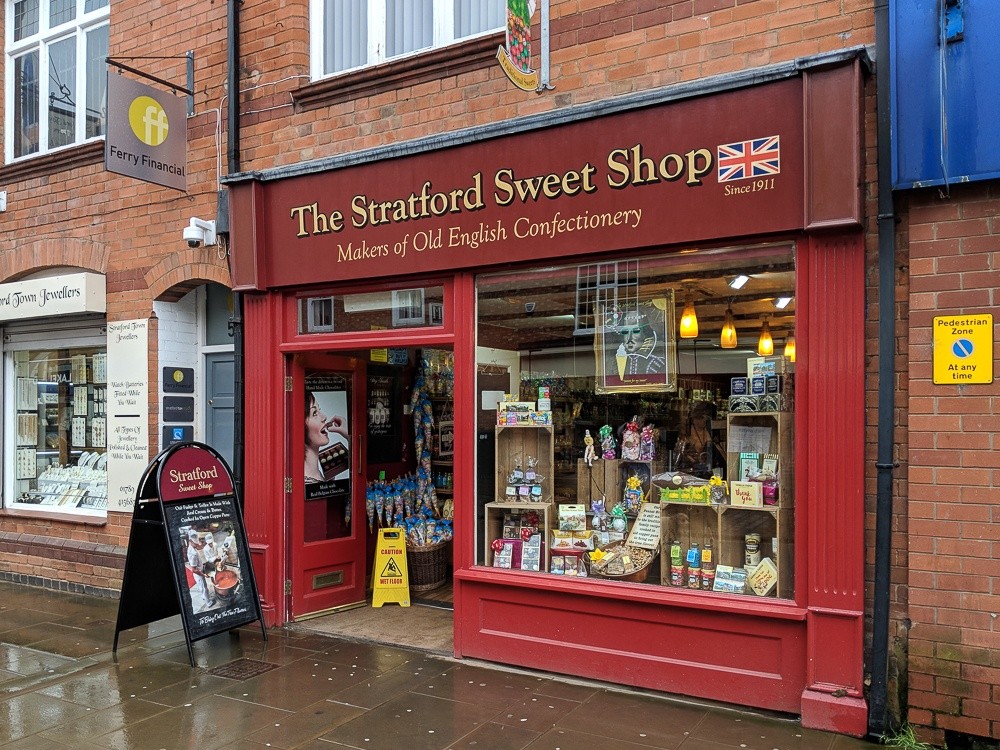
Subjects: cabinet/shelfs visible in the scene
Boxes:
[485,426,556,572]
[576,458,664,518]
[431,397,453,495]
[521,395,725,468]
[660,412,794,602]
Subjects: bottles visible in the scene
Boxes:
[670,540,715,591]
[430,471,454,489]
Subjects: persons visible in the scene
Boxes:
[680,403,717,459]
[303,389,349,481]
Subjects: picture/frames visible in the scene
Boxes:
[592,288,678,395]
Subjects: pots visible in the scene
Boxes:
[210,569,240,596]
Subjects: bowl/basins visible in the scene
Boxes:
[592,539,654,582]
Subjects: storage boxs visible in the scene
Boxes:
[749,557,777,597]
[497,387,552,426]
[729,375,783,396]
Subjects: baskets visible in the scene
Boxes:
[407,542,446,590]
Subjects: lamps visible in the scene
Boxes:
[725,274,748,290]
[758,314,774,356]
[721,304,737,348]
[679,279,698,338]
[784,322,795,356]
[770,296,793,309]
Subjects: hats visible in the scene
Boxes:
[205,534,214,545]
[188,529,199,544]
[690,401,717,421]
